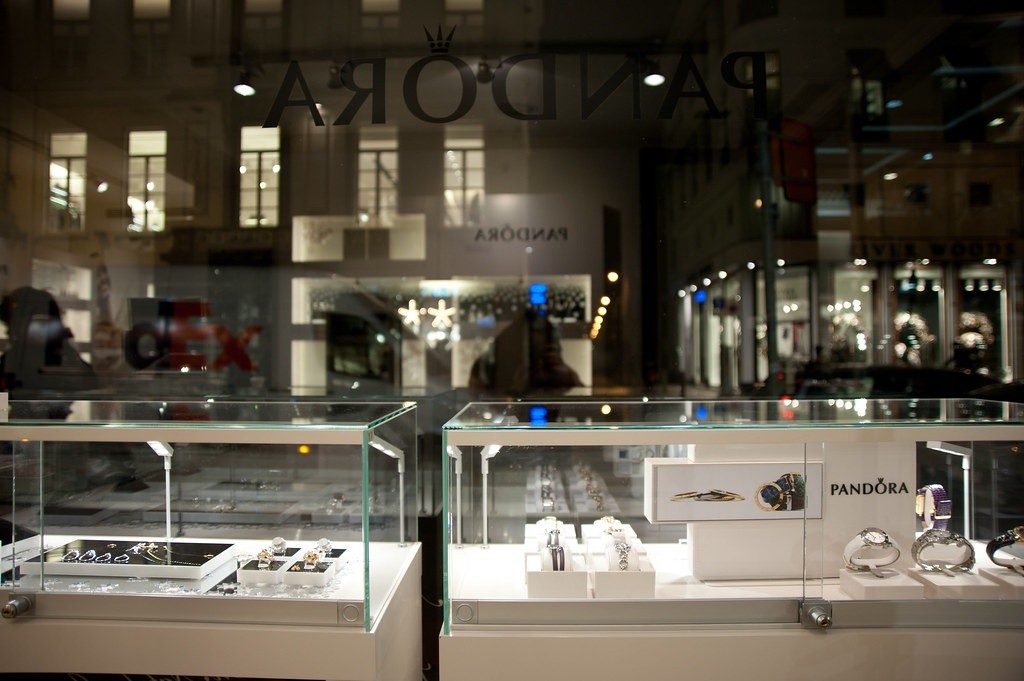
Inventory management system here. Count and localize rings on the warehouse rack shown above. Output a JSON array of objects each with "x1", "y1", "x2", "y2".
[{"x1": 62, "y1": 550, "x2": 129, "y2": 561}]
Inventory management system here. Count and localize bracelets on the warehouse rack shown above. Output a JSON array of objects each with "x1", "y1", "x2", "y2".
[
  {"x1": 579, "y1": 462, "x2": 604, "y2": 511},
  {"x1": 540, "y1": 464, "x2": 554, "y2": 511}
]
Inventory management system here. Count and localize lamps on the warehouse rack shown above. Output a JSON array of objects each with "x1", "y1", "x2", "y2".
[
  {"x1": 609, "y1": 41, "x2": 667, "y2": 102},
  {"x1": 224, "y1": 52, "x2": 274, "y2": 104}
]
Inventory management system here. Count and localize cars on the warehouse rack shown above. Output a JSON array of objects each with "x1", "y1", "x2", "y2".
[{"x1": 922, "y1": 380, "x2": 1024, "y2": 533}]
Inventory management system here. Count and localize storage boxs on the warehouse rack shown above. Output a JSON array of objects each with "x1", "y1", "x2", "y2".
[
  {"x1": 21, "y1": 540, "x2": 348, "y2": 589},
  {"x1": 523, "y1": 519, "x2": 657, "y2": 599}
]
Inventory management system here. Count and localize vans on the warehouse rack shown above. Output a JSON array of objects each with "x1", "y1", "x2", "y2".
[{"x1": 777, "y1": 364, "x2": 995, "y2": 431}]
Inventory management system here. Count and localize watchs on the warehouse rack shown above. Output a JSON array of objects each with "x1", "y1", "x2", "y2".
[
  {"x1": 916, "y1": 484, "x2": 952, "y2": 530},
  {"x1": 912, "y1": 529, "x2": 975, "y2": 573},
  {"x1": 545, "y1": 515, "x2": 565, "y2": 571},
  {"x1": 986, "y1": 527, "x2": 1024, "y2": 568},
  {"x1": 600, "y1": 515, "x2": 631, "y2": 570},
  {"x1": 844, "y1": 527, "x2": 900, "y2": 567}
]
